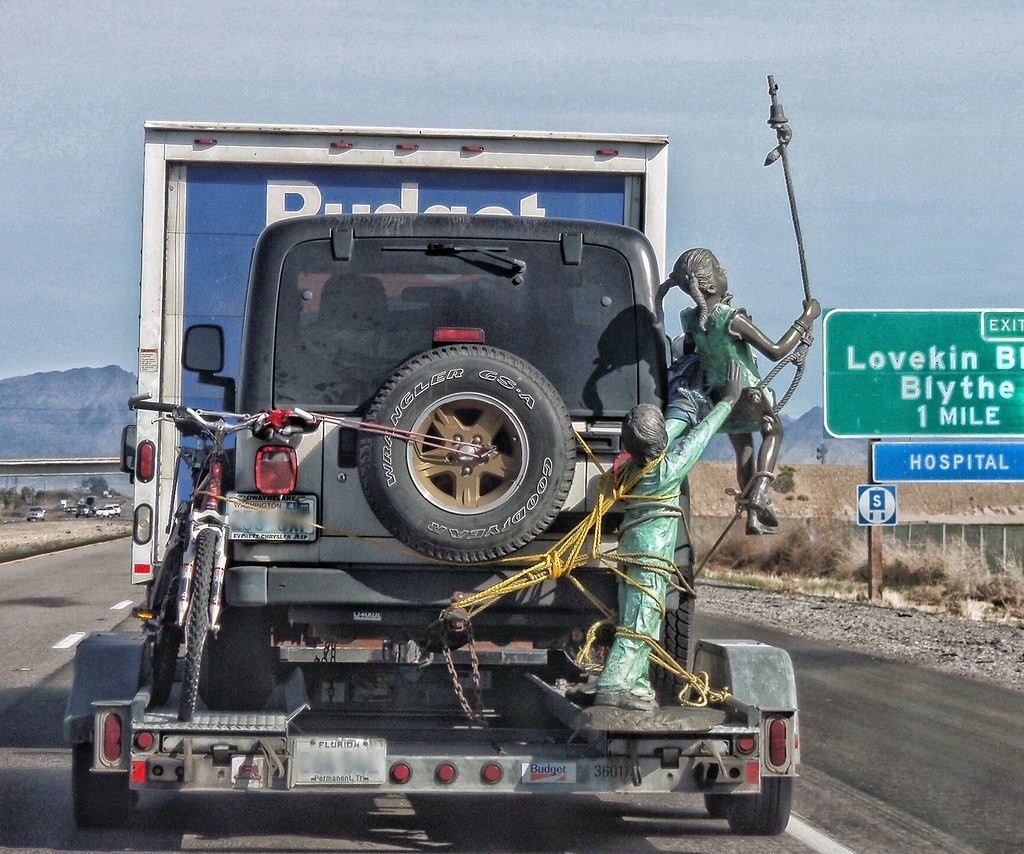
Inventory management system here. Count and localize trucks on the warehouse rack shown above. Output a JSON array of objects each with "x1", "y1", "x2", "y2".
[
  {"x1": 60, "y1": 500, "x2": 66, "y2": 510},
  {"x1": 85, "y1": 496, "x2": 97, "y2": 514}
]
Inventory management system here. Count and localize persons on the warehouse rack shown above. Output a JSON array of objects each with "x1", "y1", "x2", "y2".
[
  {"x1": 655, "y1": 248, "x2": 821, "y2": 535},
  {"x1": 592, "y1": 360, "x2": 743, "y2": 711}
]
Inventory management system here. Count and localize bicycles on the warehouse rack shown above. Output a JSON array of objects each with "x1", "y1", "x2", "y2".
[{"x1": 127, "y1": 394, "x2": 325, "y2": 723}]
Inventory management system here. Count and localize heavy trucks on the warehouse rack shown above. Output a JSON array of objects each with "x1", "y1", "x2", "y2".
[{"x1": 68, "y1": 121, "x2": 801, "y2": 836}]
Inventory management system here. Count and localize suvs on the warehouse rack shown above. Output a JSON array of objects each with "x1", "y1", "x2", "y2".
[{"x1": 223, "y1": 213, "x2": 696, "y2": 712}]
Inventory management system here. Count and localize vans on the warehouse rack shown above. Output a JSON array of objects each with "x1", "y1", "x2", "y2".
[{"x1": 75, "y1": 504, "x2": 90, "y2": 518}]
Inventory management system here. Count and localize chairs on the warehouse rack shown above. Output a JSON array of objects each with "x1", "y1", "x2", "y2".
[{"x1": 316, "y1": 273, "x2": 389, "y2": 329}]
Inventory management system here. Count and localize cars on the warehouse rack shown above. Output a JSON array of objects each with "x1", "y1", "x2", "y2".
[
  {"x1": 26, "y1": 507, "x2": 46, "y2": 521},
  {"x1": 96, "y1": 507, "x2": 111, "y2": 518},
  {"x1": 111, "y1": 504, "x2": 122, "y2": 517},
  {"x1": 64, "y1": 506, "x2": 76, "y2": 512},
  {"x1": 104, "y1": 505, "x2": 115, "y2": 517}
]
